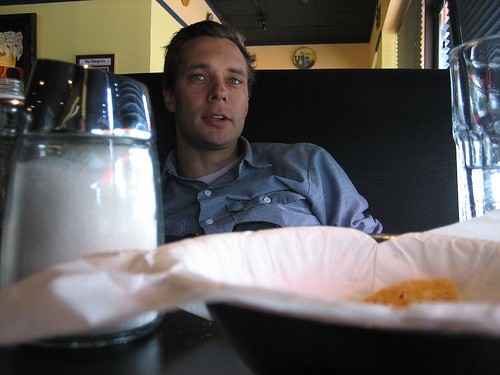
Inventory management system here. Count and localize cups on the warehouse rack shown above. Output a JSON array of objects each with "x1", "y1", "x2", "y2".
[{"x1": 446, "y1": 30, "x2": 499, "y2": 232}]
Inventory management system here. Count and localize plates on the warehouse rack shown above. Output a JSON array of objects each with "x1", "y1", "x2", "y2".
[{"x1": 201, "y1": 295, "x2": 500, "y2": 375}]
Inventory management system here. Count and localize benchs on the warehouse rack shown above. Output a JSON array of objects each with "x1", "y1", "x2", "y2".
[{"x1": 113, "y1": 67, "x2": 463, "y2": 234}]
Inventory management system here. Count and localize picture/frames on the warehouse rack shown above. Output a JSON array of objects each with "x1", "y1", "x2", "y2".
[
  {"x1": 0, "y1": 13, "x2": 38, "y2": 84},
  {"x1": 75, "y1": 54, "x2": 115, "y2": 74},
  {"x1": 291, "y1": 47, "x2": 316, "y2": 69}
]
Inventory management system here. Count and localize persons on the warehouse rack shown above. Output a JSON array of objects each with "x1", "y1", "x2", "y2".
[{"x1": 155, "y1": 17, "x2": 386, "y2": 242}]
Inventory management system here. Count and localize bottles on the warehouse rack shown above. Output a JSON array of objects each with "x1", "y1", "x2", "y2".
[{"x1": 0, "y1": 57, "x2": 172, "y2": 350}]
